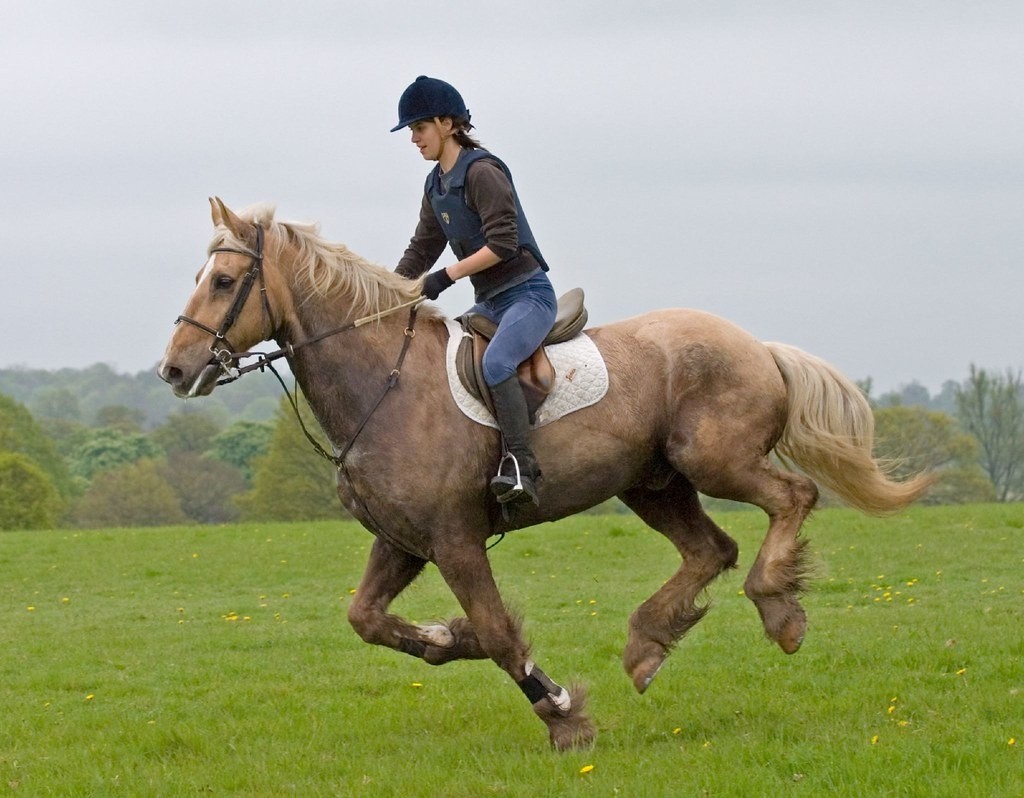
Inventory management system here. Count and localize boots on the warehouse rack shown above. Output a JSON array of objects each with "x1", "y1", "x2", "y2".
[{"x1": 490, "y1": 374, "x2": 540, "y2": 512}]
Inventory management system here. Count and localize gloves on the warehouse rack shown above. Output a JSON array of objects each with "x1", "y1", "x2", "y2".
[{"x1": 421, "y1": 268, "x2": 455, "y2": 300}]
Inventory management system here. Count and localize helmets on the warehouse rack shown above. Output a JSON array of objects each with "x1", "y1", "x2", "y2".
[{"x1": 390, "y1": 76, "x2": 472, "y2": 132}]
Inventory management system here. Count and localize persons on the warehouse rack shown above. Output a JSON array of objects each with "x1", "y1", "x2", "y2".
[{"x1": 392, "y1": 73, "x2": 558, "y2": 517}]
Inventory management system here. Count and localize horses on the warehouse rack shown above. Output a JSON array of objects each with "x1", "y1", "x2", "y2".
[{"x1": 156, "y1": 194, "x2": 940, "y2": 753}]
